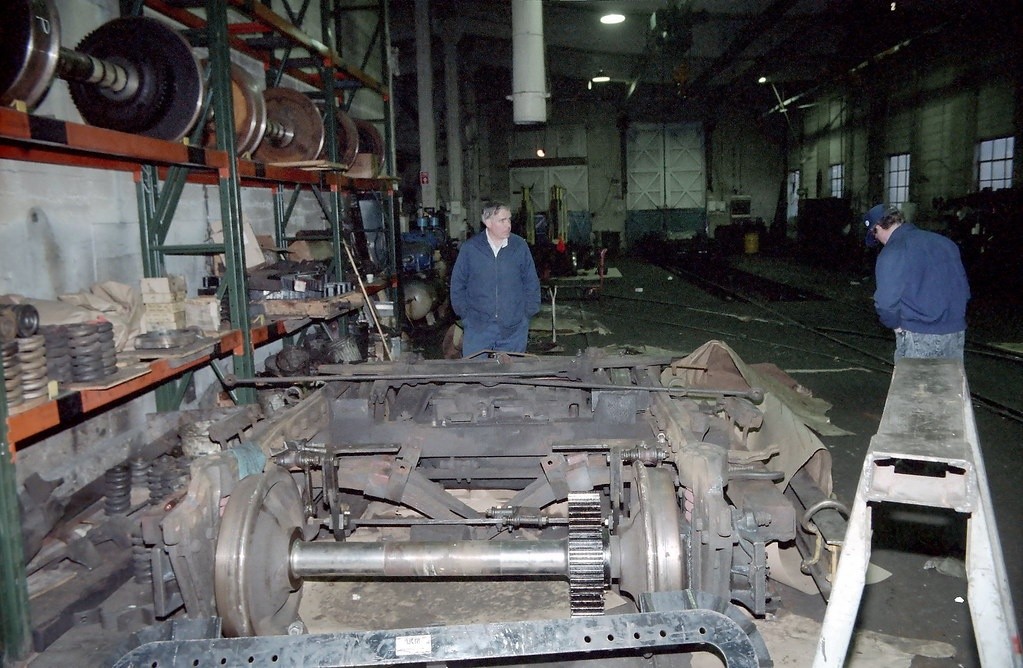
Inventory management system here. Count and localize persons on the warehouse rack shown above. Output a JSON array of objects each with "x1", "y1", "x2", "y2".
[
  {"x1": 864, "y1": 204, "x2": 971, "y2": 478},
  {"x1": 450, "y1": 201, "x2": 541, "y2": 358}
]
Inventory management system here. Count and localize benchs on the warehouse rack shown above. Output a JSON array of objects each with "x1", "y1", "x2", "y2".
[{"x1": 809, "y1": 354, "x2": 1023, "y2": 668}]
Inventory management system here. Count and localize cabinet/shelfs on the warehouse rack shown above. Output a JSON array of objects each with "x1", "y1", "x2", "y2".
[{"x1": 0, "y1": 0, "x2": 411, "y2": 668}]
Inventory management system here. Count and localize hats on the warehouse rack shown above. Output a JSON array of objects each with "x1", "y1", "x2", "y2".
[{"x1": 863, "y1": 202, "x2": 902, "y2": 246}]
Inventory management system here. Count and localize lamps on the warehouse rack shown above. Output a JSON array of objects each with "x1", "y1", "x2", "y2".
[
  {"x1": 599, "y1": 0, "x2": 627, "y2": 25},
  {"x1": 591, "y1": 66, "x2": 610, "y2": 83}
]
